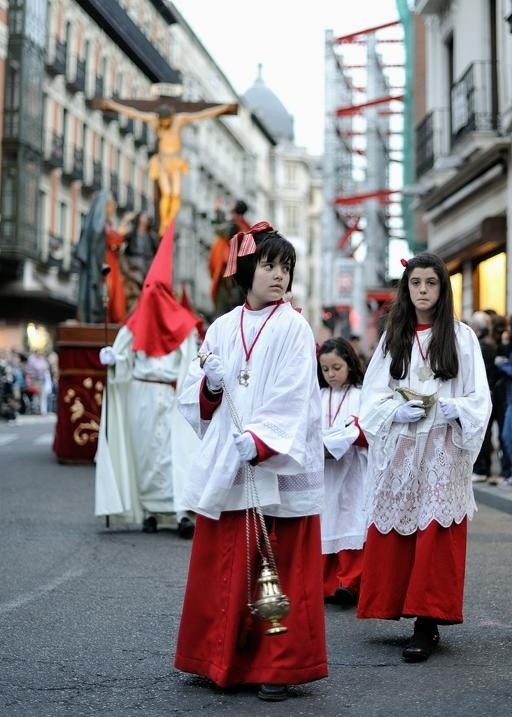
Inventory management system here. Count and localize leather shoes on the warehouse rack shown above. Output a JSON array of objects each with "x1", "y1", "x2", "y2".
[{"x1": 257, "y1": 683, "x2": 289, "y2": 702}]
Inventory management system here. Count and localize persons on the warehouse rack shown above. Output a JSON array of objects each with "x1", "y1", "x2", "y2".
[
  {"x1": 317, "y1": 336, "x2": 373, "y2": 597},
  {"x1": 174, "y1": 222, "x2": 329, "y2": 697},
  {"x1": 358, "y1": 253, "x2": 493, "y2": 663},
  {"x1": 100, "y1": 100, "x2": 231, "y2": 237},
  {"x1": 466, "y1": 309, "x2": 512, "y2": 482},
  {"x1": 96, "y1": 273, "x2": 207, "y2": 538},
  {"x1": 0, "y1": 343, "x2": 60, "y2": 422}
]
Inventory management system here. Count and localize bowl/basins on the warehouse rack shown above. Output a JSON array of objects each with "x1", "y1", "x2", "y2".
[{"x1": 391, "y1": 384, "x2": 441, "y2": 409}]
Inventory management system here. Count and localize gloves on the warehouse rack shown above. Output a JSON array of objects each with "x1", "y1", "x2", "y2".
[
  {"x1": 202, "y1": 354, "x2": 225, "y2": 392},
  {"x1": 439, "y1": 397, "x2": 459, "y2": 420},
  {"x1": 232, "y1": 430, "x2": 258, "y2": 463},
  {"x1": 393, "y1": 399, "x2": 426, "y2": 424},
  {"x1": 99, "y1": 347, "x2": 116, "y2": 367}
]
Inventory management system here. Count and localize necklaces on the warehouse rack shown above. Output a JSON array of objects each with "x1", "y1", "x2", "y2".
[
  {"x1": 415, "y1": 332, "x2": 432, "y2": 383},
  {"x1": 238, "y1": 297, "x2": 282, "y2": 386}
]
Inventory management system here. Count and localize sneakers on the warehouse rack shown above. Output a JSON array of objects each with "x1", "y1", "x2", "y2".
[
  {"x1": 142, "y1": 516, "x2": 156, "y2": 532},
  {"x1": 472, "y1": 474, "x2": 511, "y2": 490},
  {"x1": 402, "y1": 620, "x2": 439, "y2": 663},
  {"x1": 179, "y1": 516, "x2": 194, "y2": 539},
  {"x1": 335, "y1": 588, "x2": 355, "y2": 610}
]
